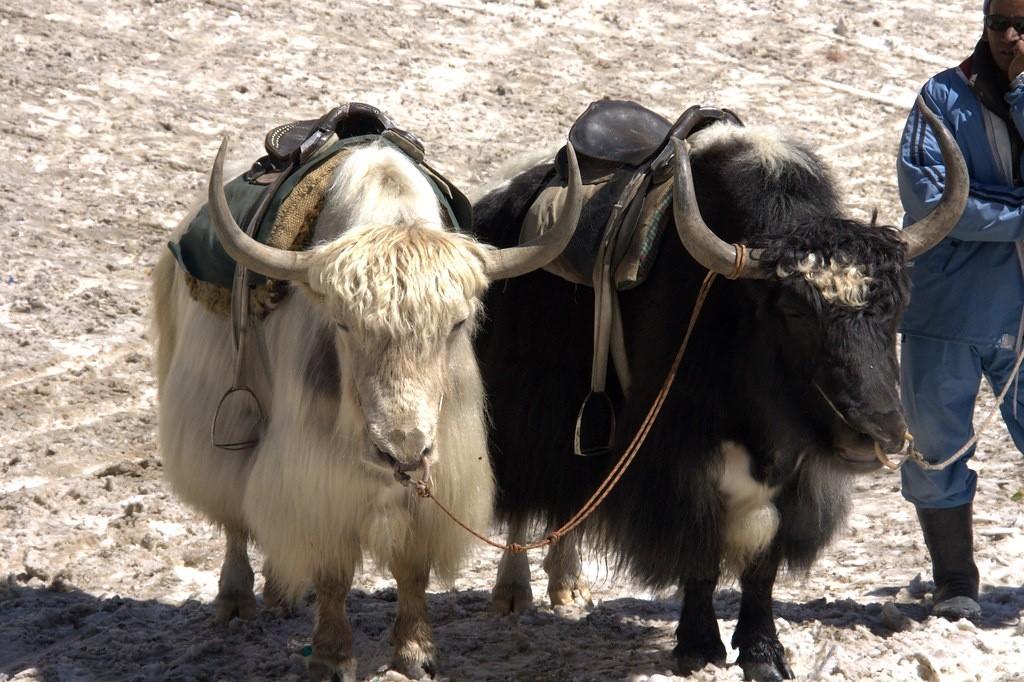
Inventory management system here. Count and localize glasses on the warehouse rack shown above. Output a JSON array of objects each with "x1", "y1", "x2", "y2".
[{"x1": 984, "y1": 15, "x2": 1024, "y2": 34}]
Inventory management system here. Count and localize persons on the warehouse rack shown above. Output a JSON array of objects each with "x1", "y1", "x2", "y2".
[{"x1": 898, "y1": 1, "x2": 1024, "y2": 621}]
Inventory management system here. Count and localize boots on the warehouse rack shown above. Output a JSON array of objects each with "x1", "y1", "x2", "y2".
[{"x1": 917, "y1": 503, "x2": 982, "y2": 620}]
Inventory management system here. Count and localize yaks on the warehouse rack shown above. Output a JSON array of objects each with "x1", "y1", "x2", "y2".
[{"x1": 154, "y1": 93, "x2": 971, "y2": 682}]
